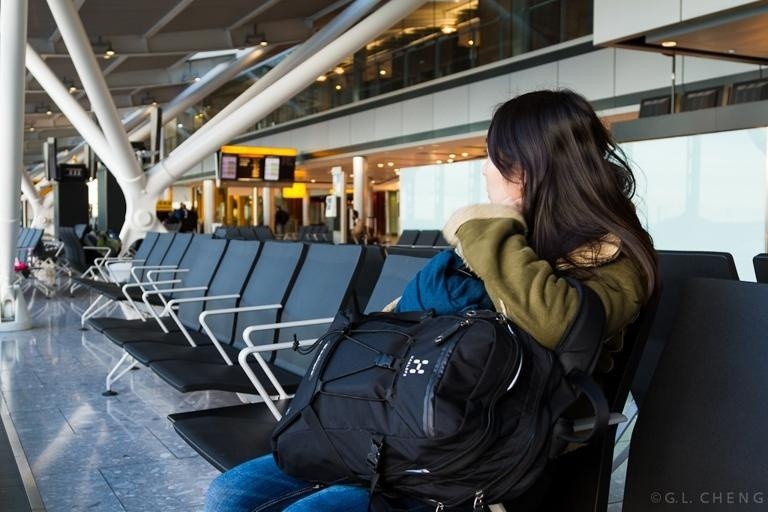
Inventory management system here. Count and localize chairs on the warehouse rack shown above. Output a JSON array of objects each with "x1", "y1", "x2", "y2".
[{"x1": 15, "y1": 219, "x2": 767, "y2": 511}]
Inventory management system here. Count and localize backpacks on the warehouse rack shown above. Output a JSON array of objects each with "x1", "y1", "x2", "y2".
[{"x1": 270, "y1": 310, "x2": 610, "y2": 511}]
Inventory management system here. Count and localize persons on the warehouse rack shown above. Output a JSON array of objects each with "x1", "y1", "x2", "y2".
[
  {"x1": 275, "y1": 204, "x2": 289, "y2": 239},
  {"x1": 201, "y1": 89, "x2": 660, "y2": 510},
  {"x1": 164, "y1": 203, "x2": 190, "y2": 226},
  {"x1": 352, "y1": 208, "x2": 363, "y2": 240}
]
